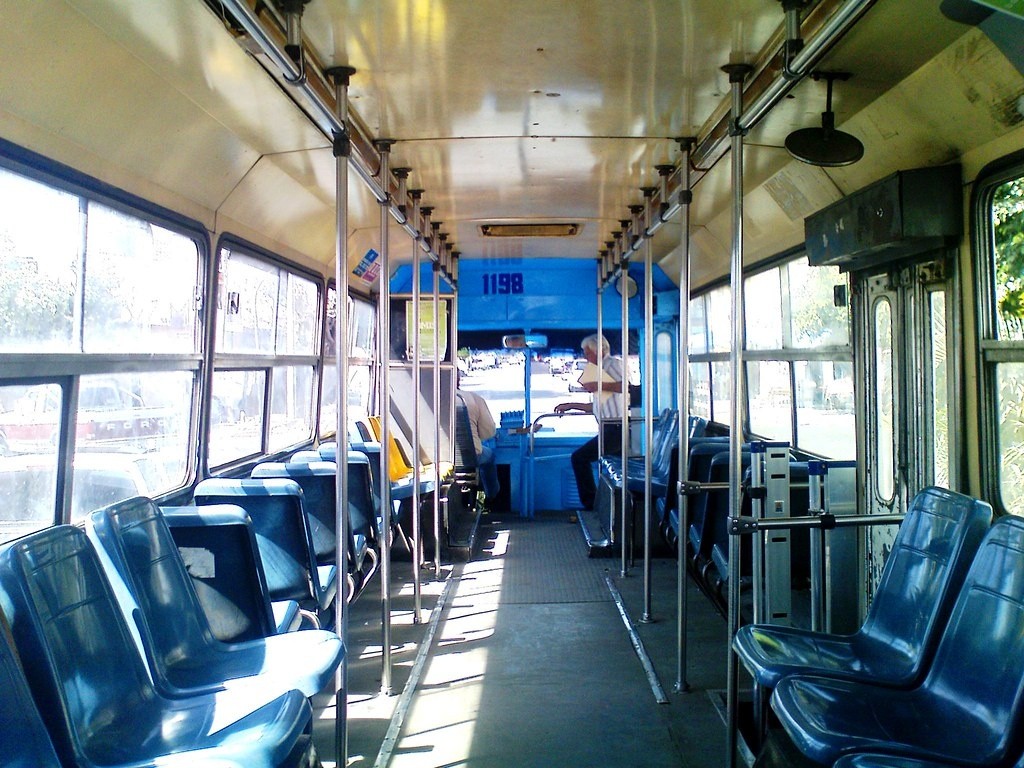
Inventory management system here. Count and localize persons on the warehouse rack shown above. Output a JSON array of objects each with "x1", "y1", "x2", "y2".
[
  {"x1": 457, "y1": 366, "x2": 500, "y2": 499},
  {"x1": 554, "y1": 335, "x2": 631, "y2": 522},
  {"x1": 506, "y1": 337, "x2": 528, "y2": 347}
]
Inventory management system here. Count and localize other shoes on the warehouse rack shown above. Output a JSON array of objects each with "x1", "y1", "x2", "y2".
[{"x1": 570, "y1": 515, "x2": 578, "y2": 523}]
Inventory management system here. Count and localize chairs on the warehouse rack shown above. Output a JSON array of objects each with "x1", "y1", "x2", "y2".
[
  {"x1": 454, "y1": 394, "x2": 492, "y2": 515},
  {"x1": 655, "y1": 437, "x2": 819, "y2": 638},
  {"x1": 158, "y1": 438, "x2": 398, "y2": 737}
]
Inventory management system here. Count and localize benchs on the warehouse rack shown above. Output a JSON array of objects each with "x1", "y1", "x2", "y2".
[
  {"x1": 347, "y1": 414, "x2": 454, "y2": 569},
  {"x1": 733, "y1": 487, "x2": 1024, "y2": 768},
  {"x1": 0, "y1": 497, "x2": 345, "y2": 768},
  {"x1": 597, "y1": 408, "x2": 705, "y2": 566}
]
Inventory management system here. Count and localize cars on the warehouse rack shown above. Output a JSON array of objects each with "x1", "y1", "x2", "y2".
[
  {"x1": 828, "y1": 376, "x2": 856, "y2": 410},
  {"x1": 561, "y1": 361, "x2": 574, "y2": 380},
  {"x1": 531, "y1": 351, "x2": 551, "y2": 364},
  {"x1": 0, "y1": 373, "x2": 188, "y2": 542},
  {"x1": 457, "y1": 352, "x2": 525, "y2": 377},
  {"x1": 567, "y1": 359, "x2": 592, "y2": 393},
  {"x1": 141, "y1": 372, "x2": 242, "y2": 420}
]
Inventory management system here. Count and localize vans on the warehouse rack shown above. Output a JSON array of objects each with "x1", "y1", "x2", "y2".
[{"x1": 550, "y1": 348, "x2": 575, "y2": 377}]
